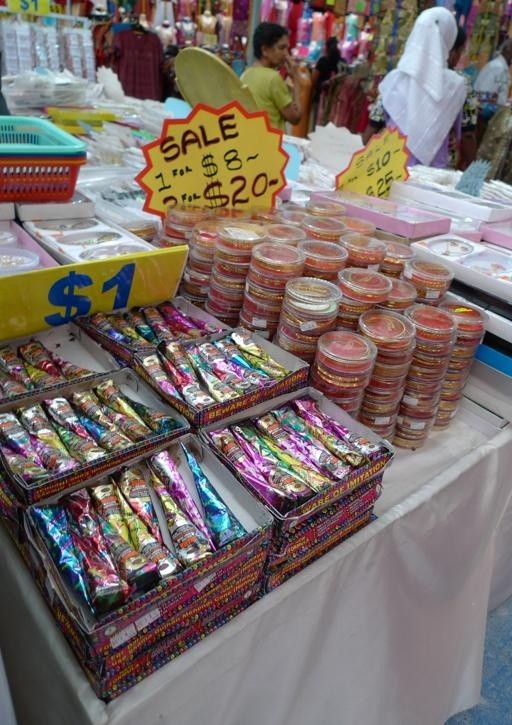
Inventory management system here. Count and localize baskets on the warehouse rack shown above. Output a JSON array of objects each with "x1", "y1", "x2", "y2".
[{"x1": 0, "y1": 113, "x2": 89, "y2": 203}]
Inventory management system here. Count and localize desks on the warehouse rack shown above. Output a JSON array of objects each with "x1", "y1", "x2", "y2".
[{"x1": 0, "y1": 88, "x2": 511, "y2": 720}]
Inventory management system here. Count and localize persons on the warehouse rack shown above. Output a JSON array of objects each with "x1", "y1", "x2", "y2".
[
  {"x1": 311, "y1": 34, "x2": 349, "y2": 98},
  {"x1": 375, "y1": 6, "x2": 474, "y2": 171},
  {"x1": 237, "y1": 21, "x2": 303, "y2": 134},
  {"x1": 475, "y1": 39, "x2": 512, "y2": 127}
]
